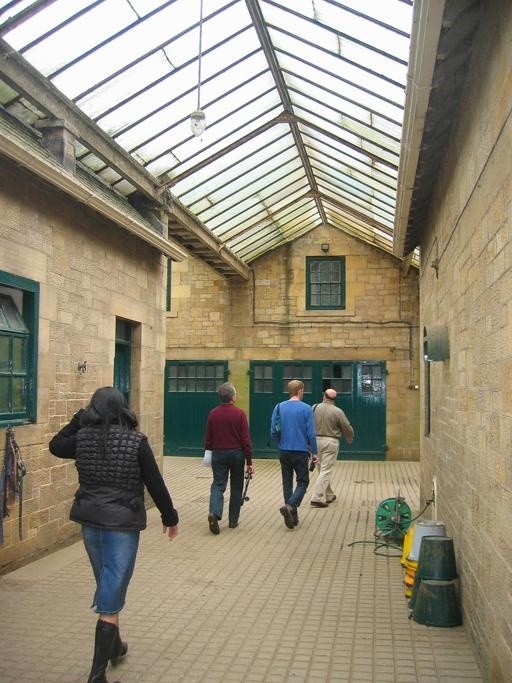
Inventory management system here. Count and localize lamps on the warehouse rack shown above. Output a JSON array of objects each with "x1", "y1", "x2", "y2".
[
  {"x1": 187, "y1": 0, "x2": 212, "y2": 138},
  {"x1": 320, "y1": 242, "x2": 330, "y2": 252}
]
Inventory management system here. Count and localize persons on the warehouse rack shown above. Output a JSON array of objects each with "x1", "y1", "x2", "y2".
[
  {"x1": 310, "y1": 388, "x2": 354, "y2": 508},
  {"x1": 205, "y1": 382, "x2": 253, "y2": 535},
  {"x1": 269, "y1": 381, "x2": 316, "y2": 528},
  {"x1": 47, "y1": 387, "x2": 179, "y2": 682}
]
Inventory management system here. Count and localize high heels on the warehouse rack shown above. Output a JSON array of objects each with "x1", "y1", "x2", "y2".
[{"x1": 110, "y1": 636, "x2": 128, "y2": 668}]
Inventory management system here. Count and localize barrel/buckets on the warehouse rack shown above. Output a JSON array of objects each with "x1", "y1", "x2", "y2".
[{"x1": 400, "y1": 520, "x2": 460, "y2": 627}]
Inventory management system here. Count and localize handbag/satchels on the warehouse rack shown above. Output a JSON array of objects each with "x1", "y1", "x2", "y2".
[{"x1": 274, "y1": 404, "x2": 281, "y2": 441}]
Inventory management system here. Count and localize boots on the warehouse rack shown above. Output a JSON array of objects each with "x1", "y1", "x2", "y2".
[{"x1": 86, "y1": 619, "x2": 119, "y2": 682}]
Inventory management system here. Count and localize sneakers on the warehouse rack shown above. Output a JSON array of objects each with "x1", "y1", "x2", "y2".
[
  {"x1": 280, "y1": 506, "x2": 295, "y2": 529},
  {"x1": 229, "y1": 521, "x2": 240, "y2": 528},
  {"x1": 291, "y1": 506, "x2": 297, "y2": 526},
  {"x1": 310, "y1": 500, "x2": 329, "y2": 508},
  {"x1": 327, "y1": 494, "x2": 337, "y2": 503},
  {"x1": 207, "y1": 512, "x2": 220, "y2": 534}
]
